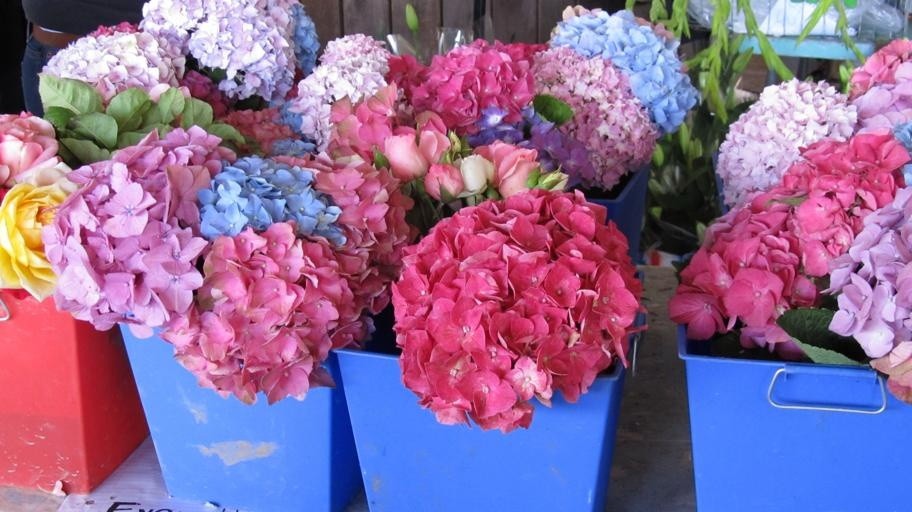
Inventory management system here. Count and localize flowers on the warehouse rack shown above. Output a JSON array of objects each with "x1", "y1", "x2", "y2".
[
  {"x1": 38, "y1": 22, "x2": 246, "y2": 168},
  {"x1": 846, "y1": 38, "x2": 912, "y2": 117},
  {"x1": 47, "y1": 127, "x2": 420, "y2": 407},
  {"x1": 672, "y1": 119, "x2": 912, "y2": 406},
  {"x1": 423, "y1": 42, "x2": 531, "y2": 148},
  {"x1": 715, "y1": 76, "x2": 857, "y2": 207},
  {"x1": 0, "y1": 110, "x2": 82, "y2": 303},
  {"x1": 392, "y1": 188, "x2": 650, "y2": 433},
  {"x1": 299, "y1": 30, "x2": 423, "y2": 148},
  {"x1": 141, "y1": 0, "x2": 320, "y2": 112},
  {"x1": 535, "y1": 0, "x2": 698, "y2": 192}
]
